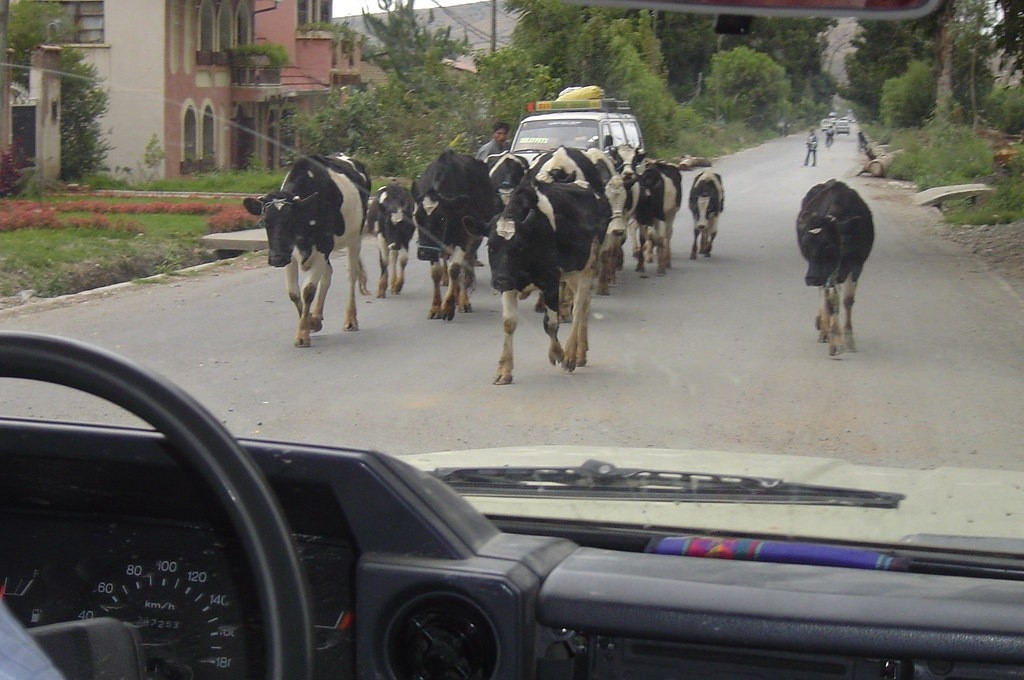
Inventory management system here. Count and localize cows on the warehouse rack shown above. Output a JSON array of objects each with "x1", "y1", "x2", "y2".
[
  {"x1": 241, "y1": 143, "x2": 726, "y2": 386},
  {"x1": 795, "y1": 178, "x2": 875, "y2": 356}
]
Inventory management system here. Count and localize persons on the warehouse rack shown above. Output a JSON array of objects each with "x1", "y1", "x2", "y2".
[
  {"x1": 804, "y1": 127, "x2": 817, "y2": 166},
  {"x1": 826, "y1": 125, "x2": 834, "y2": 148},
  {"x1": 476, "y1": 123, "x2": 511, "y2": 172},
  {"x1": 858, "y1": 131, "x2": 867, "y2": 150}
]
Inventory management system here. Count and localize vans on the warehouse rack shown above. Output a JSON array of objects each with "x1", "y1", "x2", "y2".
[
  {"x1": 509, "y1": 112, "x2": 647, "y2": 169},
  {"x1": 836, "y1": 121, "x2": 849, "y2": 135}
]
